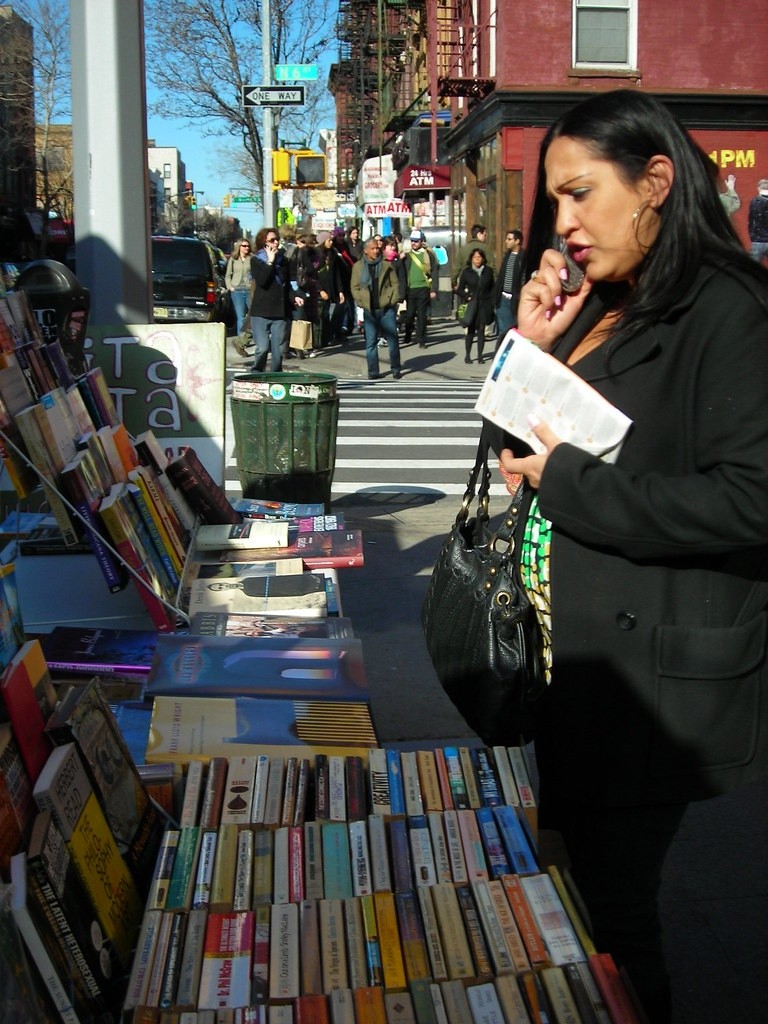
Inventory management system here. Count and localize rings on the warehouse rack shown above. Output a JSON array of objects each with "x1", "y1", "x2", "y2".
[{"x1": 531, "y1": 271, "x2": 543, "y2": 285}]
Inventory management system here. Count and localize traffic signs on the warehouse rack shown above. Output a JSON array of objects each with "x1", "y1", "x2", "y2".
[{"x1": 242, "y1": 84, "x2": 306, "y2": 108}]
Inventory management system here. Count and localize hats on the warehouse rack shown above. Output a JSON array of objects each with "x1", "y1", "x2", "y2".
[
  {"x1": 316, "y1": 232, "x2": 331, "y2": 245},
  {"x1": 333, "y1": 227, "x2": 345, "y2": 237},
  {"x1": 410, "y1": 230, "x2": 425, "y2": 241}
]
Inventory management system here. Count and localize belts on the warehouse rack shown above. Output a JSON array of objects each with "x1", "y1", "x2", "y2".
[{"x1": 501, "y1": 292, "x2": 512, "y2": 301}]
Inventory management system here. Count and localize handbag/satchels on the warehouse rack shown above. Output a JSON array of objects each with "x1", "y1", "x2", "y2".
[
  {"x1": 461, "y1": 301, "x2": 477, "y2": 328},
  {"x1": 424, "y1": 272, "x2": 432, "y2": 287},
  {"x1": 296, "y1": 266, "x2": 309, "y2": 287},
  {"x1": 419, "y1": 432, "x2": 549, "y2": 747},
  {"x1": 289, "y1": 304, "x2": 313, "y2": 350}
]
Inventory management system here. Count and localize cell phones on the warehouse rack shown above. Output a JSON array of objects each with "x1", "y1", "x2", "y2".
[{"x1": 560, "y1": 244, "x2": 586, "y2": 292}]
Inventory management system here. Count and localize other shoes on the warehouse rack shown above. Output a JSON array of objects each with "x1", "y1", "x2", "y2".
[
  {"x1": 420, "y1": 344, "x2": 427, "y2": 349},
  {"x1": 478, "y1": 358, "x2": 485, "y2": 364},
  {"x1": 465, "y1": 358, "x2": 473, "y2": 364},
  {"x1": 304, "y1": 349, "x2": 326, "y2": 358},
  {"x1": 404, "y1": 333, "x2": 411, "y2": 343},
  {"x1": 294, "y1": 350, "x2": 306, "y2": 359},
  {"x1": 283, "y1": 352, "x2": 295, "y2": 360},
  {"x1": 231, "y1": 339, "x2": 248, "y2": 356}
]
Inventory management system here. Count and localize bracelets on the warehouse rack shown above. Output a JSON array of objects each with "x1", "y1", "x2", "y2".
[{"x1": 267, "y1": 260, "x2": 272, "y2": 264}]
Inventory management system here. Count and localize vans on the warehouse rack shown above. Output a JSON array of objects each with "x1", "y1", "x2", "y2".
[{"x1": 152, "y1": 233, "x2": 230, "y2": 323}]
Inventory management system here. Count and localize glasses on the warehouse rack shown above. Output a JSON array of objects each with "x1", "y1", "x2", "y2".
[
  {"x1": 411, "y1": 240, "x2": 418, "y2": 242},
  {"x1": 241, "y1": 245, "x2": 249, "y2": 247},
  {"x1": 266, "y1": 238, "x2": 279, "y2": 243}
]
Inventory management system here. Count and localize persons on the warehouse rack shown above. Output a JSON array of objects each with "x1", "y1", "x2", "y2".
[
  {"x1": 189, "y1": 222, "x2": 529, "y2": 380},
  {"x1": 486, "y1": 88, "x2": 768, "y2": 1024},
  {"x1": 717, "y1": 172, "x2": 768, "y2": 266},
  {"x1": 205, "y1": 573, "x2": 325, "y2": 598}
]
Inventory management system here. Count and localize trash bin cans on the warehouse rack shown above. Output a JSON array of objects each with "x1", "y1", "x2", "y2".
[{"x1": 231, "y1": 368, "x2": 343, "y2": 516}]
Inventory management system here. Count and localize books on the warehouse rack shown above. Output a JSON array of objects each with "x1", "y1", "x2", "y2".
[{"x1": 0, "y1": 289, "x2": 643, "y2": 1024}]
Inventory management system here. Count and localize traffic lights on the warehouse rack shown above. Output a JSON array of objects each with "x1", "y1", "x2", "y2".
[
  {"x1": 191, "y1": 197, "x2": 196, "y2": 211},
  {"x1": 294, "y1": 155, "x2": 327, "y2": 187}
]
